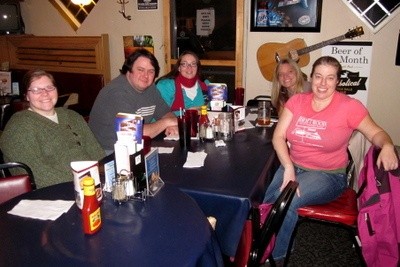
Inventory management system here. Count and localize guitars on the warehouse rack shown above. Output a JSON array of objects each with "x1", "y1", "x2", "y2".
[{"x1": 256, "y1": 25, "x2": 365, "y2": 82}]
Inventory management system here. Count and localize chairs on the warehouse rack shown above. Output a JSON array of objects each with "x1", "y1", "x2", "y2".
[
  {"x1": 233, "y1": 180, "x2": 300, "y2": 267},
  {"x1": 283, "y1": 131, "x2": 366, "y2": 267},
  {"x1": 0, "y1": 163, "x2": 37, "y2": 205}
]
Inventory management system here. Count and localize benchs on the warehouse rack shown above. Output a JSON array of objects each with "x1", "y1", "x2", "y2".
[{"x1": 9, "y1": 69, "x2": 107, "y2": 127}]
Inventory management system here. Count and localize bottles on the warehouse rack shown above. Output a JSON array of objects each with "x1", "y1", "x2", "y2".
[
  {"x1": 198, "y1": 106, "x2": 209, "y2": 143},
  {"x1": 111, "y1": 173, "x2": 136, "y2": 205},
  {"x1": 81, "y1": 177, "x2": 102, "y2": 234}
]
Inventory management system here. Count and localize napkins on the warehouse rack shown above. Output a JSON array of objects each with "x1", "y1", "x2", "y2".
[
  {"x1": 234, "y1": 113, "x2": 277, "y2": 131},
  {"x1": 151, "y1": 147, "x2": 175, "y2": 154},
  {"x1": 215, "y1": 139, "x2": 227, "y2": 147},
  {"x1": 7, "y1": 199, "x2": 75, "y2": 220},
  {"x1": 164, "y1": 134, "x2": 178, "y2": 141},
  {"x1": 184, "y1": 151, "x2": 207, "y2": 168}
]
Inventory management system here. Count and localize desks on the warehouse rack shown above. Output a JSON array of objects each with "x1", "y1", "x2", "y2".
[
  {"x1": 99, "y1": 118, "x2": 277, "y2": 267},
  {"x1": 0, "y1": 181, "x2": 227, "y2": 267}
]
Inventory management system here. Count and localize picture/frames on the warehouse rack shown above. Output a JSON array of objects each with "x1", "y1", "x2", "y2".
[{"x1": 250, "y1": 0, "x2": 324, "y2": 32}]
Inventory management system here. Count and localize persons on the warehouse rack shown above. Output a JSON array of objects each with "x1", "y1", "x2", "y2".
[
  {"x1": 271, "y1": 59, "x2": 312, "y2": 120},
  {"x1": 0, "y1": 67, "x2": 106, "y2": 190},
  {"x1": 150, "y1": 50, "x2": 211, "y2": 124},
  {"x1": 88, "y1": 48, "x2": 179, "y2": 150},
  {"x1": 264, "y1": 56, "x2": 399, "y2": 262}
]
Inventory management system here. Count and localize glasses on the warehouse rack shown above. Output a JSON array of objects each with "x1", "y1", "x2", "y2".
[
  {"x1": 180, "y1": 62, "x2": 198, "y2": 67},
  {"x1": 26, "y1": 86, "x2": 56, "y2": 95}
]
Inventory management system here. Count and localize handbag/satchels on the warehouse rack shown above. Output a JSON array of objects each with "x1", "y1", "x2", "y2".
[{"x1": 259, "y1": 202, "x2": 275, "y2": 263}]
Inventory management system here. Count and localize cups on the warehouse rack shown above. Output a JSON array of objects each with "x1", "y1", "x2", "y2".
[
  {"x1": 184, "y1": 108, "x2": 198, "y2": 137},
  {"x1": 258, "y1": 102, "x2": 271, "y2": 125},
  {"x1": 178, "y1": 114, "x2": 191, "y2": 153},
  {"x1": 217, "y1": 112, "x2": 233, "y2": 141}
]
